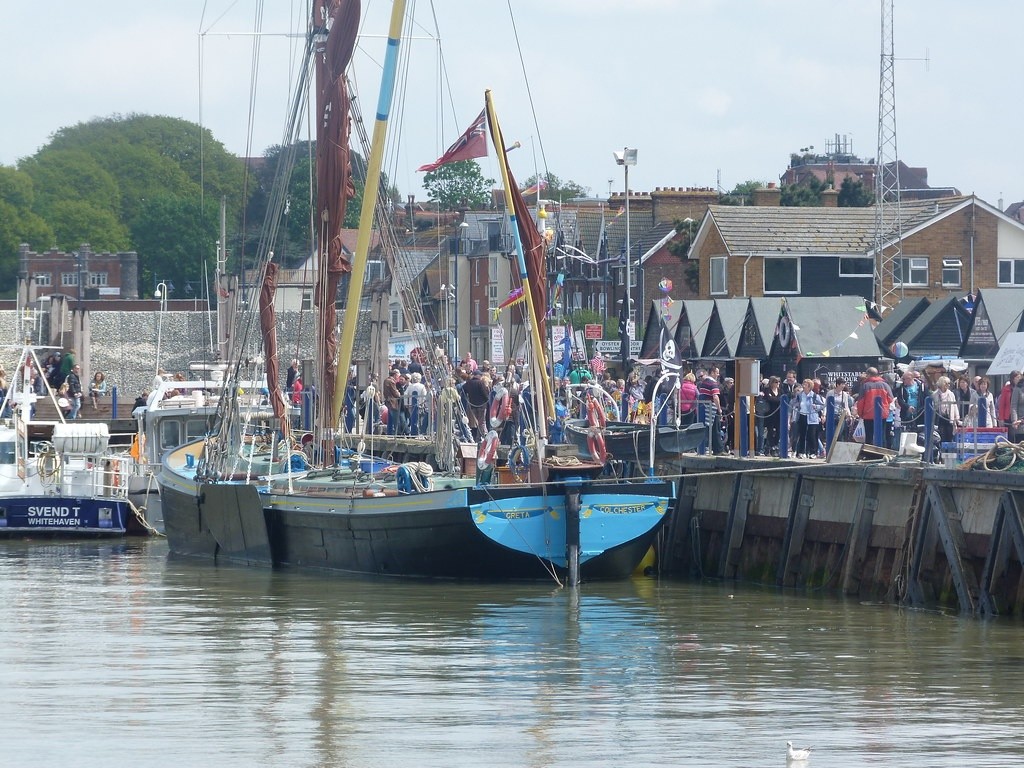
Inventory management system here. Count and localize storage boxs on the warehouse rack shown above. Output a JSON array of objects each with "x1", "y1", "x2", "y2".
[
  {"x1": 957, "y1": 443, "x2": 997, "y2": 460},
  {"x1": 350, "y1": 460, "x2": 390, "y2": 473},
  {"x1": 956, "y1": 428, "x2": 1008, "y2": 443}
]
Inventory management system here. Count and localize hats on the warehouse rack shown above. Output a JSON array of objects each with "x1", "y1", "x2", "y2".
[
  {"x1": 474, "y1": 370, "x2": 483, "y2": 376},
  {"x1": 55, "y1": 352, "x2": 60, "y2": 356}
]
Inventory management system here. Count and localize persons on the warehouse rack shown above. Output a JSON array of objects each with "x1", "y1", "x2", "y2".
[
  {"x1": 131, "y1": 369, "x2": 187, "y2": 432},
  {"x1": 523, "y1": 352, "x2": 664, "y2": 445},
  {"x1": 343, "y1": 357, "x2": 441, "y2": 435},
  {"x1": 435, "y1": 352, "x2": 524, "y2": 442},
  {"x1": 0, "y1": 349, "x2": 107, "y2": 420},
  {"x1": 923, "y1": 361, "x2": 1024, "y2": 452},
  {"x1": 681, "y1": 365, "x2": 928, "y2": 458},
  {"x1": 287, "y1": 359, "x2": 317, "y2": 407}
]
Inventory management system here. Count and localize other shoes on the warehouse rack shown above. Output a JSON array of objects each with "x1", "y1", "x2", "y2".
[
  {"x1": 809, "y1": 454, "x2": 816, "y2": 459},
  {"x1": 713, "y1": 445, "x2": 797, "y2": 458},
  {"x1": 799, "y1": 453, "x2": 806, "y2": 459},
  {"x1": 817, "y1": 450, "x2": 826, "y2": 458}
]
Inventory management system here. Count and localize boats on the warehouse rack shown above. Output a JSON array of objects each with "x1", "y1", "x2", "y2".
[
  {"x1": 114, "y1": 361, "x2": 300, "y2": 535},
  {"x1": 0, "y1": 339, "x2": 136, "y2": 534}
]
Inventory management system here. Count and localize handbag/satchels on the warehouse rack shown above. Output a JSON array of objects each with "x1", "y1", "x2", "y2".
[
  {"x1": 836, "y1": 401, "x2": 846, "y2": 422},
  {"x1": 853, "y1": 417, "x2": 866, "y2": 442}
]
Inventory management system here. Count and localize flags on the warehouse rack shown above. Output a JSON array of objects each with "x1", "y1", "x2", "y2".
[{"x1": 416, "y1": 107, "x2": 487, "y2": 172}]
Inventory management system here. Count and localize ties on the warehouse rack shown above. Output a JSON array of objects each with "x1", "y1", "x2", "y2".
[{"x1": 789, "y1": 384, "x2": 793, "y2": 392}]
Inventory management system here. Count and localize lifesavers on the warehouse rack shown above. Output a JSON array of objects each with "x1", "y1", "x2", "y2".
[
  {"x1": 489, "y1": 387, "x2": 509, "y2": 428},
  {"x1": 477, "y1": 429, "x2": 498, "y2": 469},
  {"x1": 113, "y1": 460, "x2": 119, "y2": 486},
  {"x1": 585, "y1": 395, "x2": 606, "y2": 430},
  {"x1": 587, "y1": 426, "x2": 606, "y2": 465},
  {"x1": 404, "y1": 382, "x2": 427, "y2": 408}
]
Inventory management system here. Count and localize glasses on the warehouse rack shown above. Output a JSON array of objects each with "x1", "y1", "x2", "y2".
[
  {"x1": 480, "y1": 374, "x2": 482, "y2": 376},
  {"x1": 697, "y1": 374, "x2": 705, "y2": 379},
  {"x1": 729, "y1": 382, "x2": 734, "y2": 384},
  {"x1": 902, "y1": 378, "x2": 910, "y2": 380},
  {"x1": 773, "y1": 381, "x2": 780, "y2": 383},
  {"x1": 914, "y1": 376, "x2": 920, "y2": 378}
]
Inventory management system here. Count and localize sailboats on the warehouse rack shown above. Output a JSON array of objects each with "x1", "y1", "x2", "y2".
[{"x1": 156, "y1": 0, "x2": 679, "y2": 589}]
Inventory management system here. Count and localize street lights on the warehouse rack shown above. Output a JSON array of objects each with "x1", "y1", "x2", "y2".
[
  {"x1": 613, "y1": 146, "x2": 639, "y2": 389},
  {"x1": 453, "y1": 217, "x2": 471, "y2": 372},
  {"x1": 601, "y1": 230, "x2": 608, "y2": 343}
]
